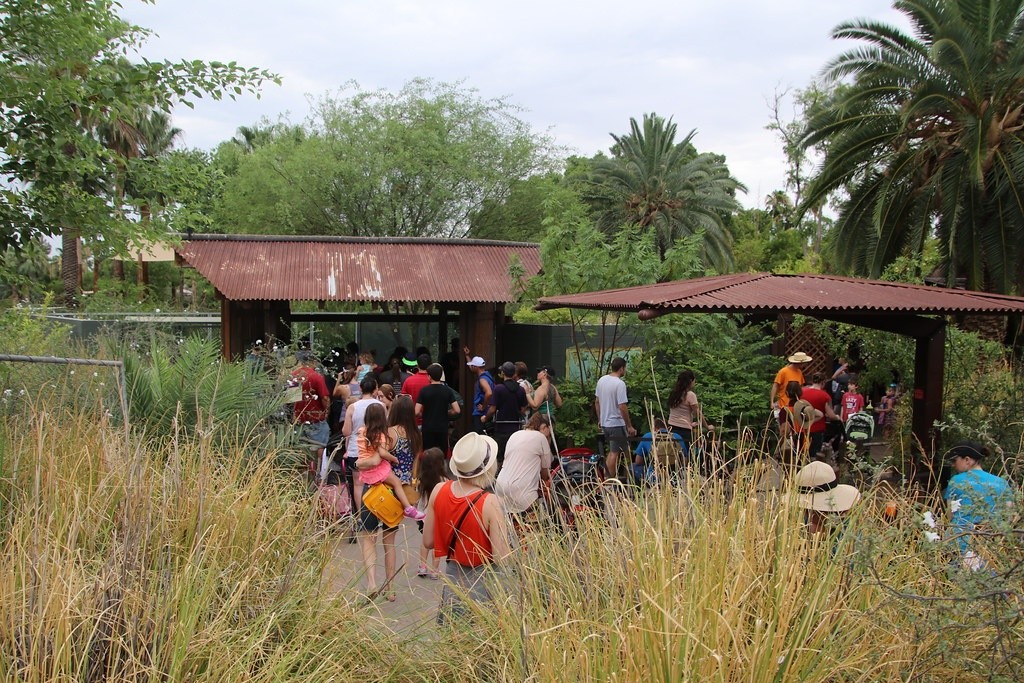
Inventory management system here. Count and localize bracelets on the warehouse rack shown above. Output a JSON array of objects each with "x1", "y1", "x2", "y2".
[
  {"x1": 355, "y1": 463, "x2": 356, "y2": 467},
  {"x1": 465, "y1": 354, "x2": 469, "y2": 356}
]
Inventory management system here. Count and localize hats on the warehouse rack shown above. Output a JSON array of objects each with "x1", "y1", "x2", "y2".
[
  {"x1": 788, "y1": 352, "x2": 812, "y2": 363},
  {"x1": 782, "y1": 461, "x2": 861, "y2": 512},
  {"x1": 466, "y1": 356, "x2": 486, "y2": 366},
  {"x1": 536, "y1": 365, "x2": 555, "y2": 377},
  {"x1": 295, "y1": 350, "x2": 321, "y2": 362},
  {"x1": 794, "y1": 399, "x2": 815, "y2": 429},
  {"x1": 402, "y1": 353, "x2": 418, "y2": 366},
  {"x1": 942, "y1": 441, "x2": 985, "y2": 460},
  {"x1": 449, "y1": 432, "x2": 499, "y2": 478},
  {"x1": 499, "y1": 361, "x2": 516, "y2": 375}
]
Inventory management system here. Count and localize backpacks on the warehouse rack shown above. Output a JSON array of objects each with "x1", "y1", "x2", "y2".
[{"x1": 651, "y1": 434, "x2": 682, "y2": 466}]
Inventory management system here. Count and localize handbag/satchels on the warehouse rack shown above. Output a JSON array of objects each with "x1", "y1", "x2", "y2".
[
  {"x1": 364, "y1": 484, "x2": 405, "y2": 529},
  {"x1": 320, "y1": 483, "x2": 352, "y2": 517}
]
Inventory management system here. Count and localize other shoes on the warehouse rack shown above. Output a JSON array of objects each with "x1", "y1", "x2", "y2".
[
  {"x1": 416, "y1": 565, "x2": 428, "y2": 576},
  {"x1": 382, "y1": 590, "x2": 398, "y2": 602},
  {"x1": 431, "y1": 568, "x2": 440, "y2": 580},
  {"x1": 348, "y1": 535, "x2": 357, "y2": 545},
  {"x1": 366, "y1": 590, "x2": 378, "y2": 604}
]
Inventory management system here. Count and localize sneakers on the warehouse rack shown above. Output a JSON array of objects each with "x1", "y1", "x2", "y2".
[{"x1": 403, "y1": 505, "x2": 426, "y2": 520}]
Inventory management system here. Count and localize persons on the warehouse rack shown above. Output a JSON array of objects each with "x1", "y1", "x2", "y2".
[
  {"x1": 877, "y1": 384, "x2": 898, "y2": 441},
  {"x1": 782, "y1": 460, "x2": 861, "y2": 542},
  {"x1": 771, "y1": 352, "x2": 813, "y2": 458},
  {"x1": 840, "y1": 376, "x2": 865, "y2": 424},
  {"x1": 595, "y1": 357, "x2": 637, "y2": 476},
  {"x1": 947, "y1": 442, "x2": 1015, "y2": 577},
  {"x1": 354, "y1": 394, "x2": 421, "y2": 602},
  {"x1": 800, "y1": 371, "x2": 836, "y2": 457},
  {"x1": 668, "y1": 370, "x2": 714, "y2": 451},
  {"x1": 248, "y1": 338, "x2": 562, "y2": 546},
  {"x1": 779, "y1": 381, "x2": 806, "y2": 466},
  {"x1": 416, "y1": 448, "x2": 451, "y2": 579},
  {"x1": 496, "y1": 412, "x2": 555, "y2": 518},
  {"x1": 630, "y1": 418, "x2": 688, "y2": 488},
  {"x1": 423, "y1": 431, "x2": 517, "y2": 633},
  {"x1": 831, "y1": 361, "x2": 859, "y2": 415},
  {"x1": 354, "y1": 403, "x2": 426, "y2": 525}
]
